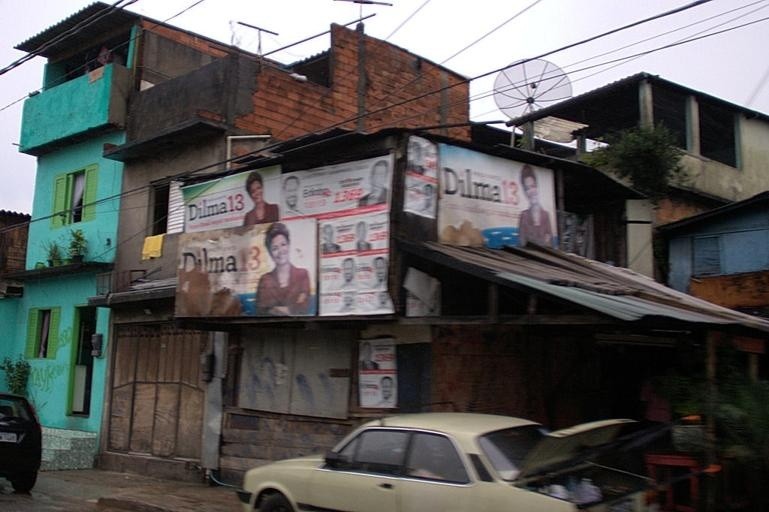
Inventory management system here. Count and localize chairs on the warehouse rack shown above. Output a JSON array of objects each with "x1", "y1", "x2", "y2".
[
  {"x1": 0, "y1": 406, "x2": 13, "y2": 418},
  {"x1": 398, "y1": 437, "x2": 432, "y2": 470}
]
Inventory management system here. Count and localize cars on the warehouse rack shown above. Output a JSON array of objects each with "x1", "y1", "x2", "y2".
[
  {"x1": 0, "y1": 393, "x2": 41, "y2": 492},
  {"x1": 238, "y1": 411, "x2": 660, "y2": 512}
]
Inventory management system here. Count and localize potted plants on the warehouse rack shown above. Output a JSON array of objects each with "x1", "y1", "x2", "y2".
[{"x1": 41, "y1": 229, "x2": 87, "y2": 267}]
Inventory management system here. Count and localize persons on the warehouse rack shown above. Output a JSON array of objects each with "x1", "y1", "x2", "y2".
[
  {"x1": 282, "y1": 176, "x2": 299, "y2": 208},
  {"x1": 321, "y1": 225, "x2": 340, "y2": 254},
  {"x1": 520, "y1": 165, "x2": 552, "y2": 247},
  {"x1": 359, "y1": 160, "x2": 387, "y2": 206},
  {"x1": 355, "y1": 221, "x2": 371, "y2": 251},
  {"x1": 339, "y1": 256, "x2": 389, "y2": 313},
  {"x1": 243, "y1": 172, "x2": 278, "y2": 225},
  {"x1": 257, "y1": 223, "x2": 310, "y2": 314},
  {"x1": 641, "y1": 380, "x2": 673, "y2": 429},
  {"x1": 377, "y1": 377, "x2": 394, "y2": 407},
  {"x1": 359, "y1": 342, "x2": 378, "y2": 370},
  {"x1": 419, "y1": 184, "x2": 436, "y2": 213},
  {"x1": 406, "y1": 142, "x2": 424, "y2": 175}
]
